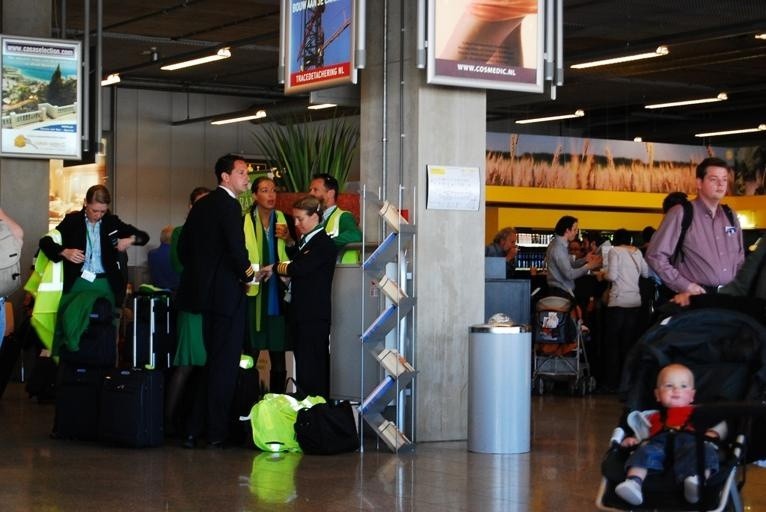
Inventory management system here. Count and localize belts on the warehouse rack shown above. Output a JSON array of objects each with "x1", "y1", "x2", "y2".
[{"x1": 698, "y1": 283, "x2": 726, "y2": 293}]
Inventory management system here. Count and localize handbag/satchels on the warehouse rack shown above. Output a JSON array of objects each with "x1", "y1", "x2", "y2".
[
  {"x1": 640, "y1": 277, "x2": 662, "y2": 309},
  {"x1": 237, "y1": 378, "x2": 327, "y2": 454},
  {"x1": 292, "y1": 399, "x2": 359, "y2": 457}
]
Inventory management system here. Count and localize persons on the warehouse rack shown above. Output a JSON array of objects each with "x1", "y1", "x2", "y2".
[
  {"x1": 238, "y1": 176, "x2": 292, "y2": 400},
  {"x1": 175, "y1": 153, "x2": 257, "y2": 451},
  {"x1": 597, "y1": 227, "x2": 644, "y2": 390},
  {"x1": 486, "y1": 227, "x2": 521, "y2": 279},
  {"x1": 307, "y1": 172, "x2": 364, "y2": 264},
  {"x1": 40, "y1": 185, "x2": 151, "y2": 364},
  {"x1": 535, "y1": 207, "x2": 605, "y2": 373},
  {"x1": 643, "y1": 156, "x2": 747, "y2": 306},
  {"x1": 568, "y1": 240, "x2": 584, "y2": 261},
  {"x1": 147, "y1": 225, "x2": 182, "y2": 291},
  {"x1": 580, "y1": 229, "x2": 606, "y2": 318},
  {"x1": 255, "y1": 193, "x2": 338, "y2": 399},
  {"x1": 614, "y1": 362, "x2": 729, "y2": 507},
  {"x1": 640, "y1": 225, "x2": 662, "y2": 284},
  {"x1": 0, "y1": 208, "x2": 25, "y2": 350},
  {"x1": 164, "y1": 186, "x2": 219, "y2": 437}
]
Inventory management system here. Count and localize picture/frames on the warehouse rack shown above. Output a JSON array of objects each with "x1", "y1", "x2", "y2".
[
  {"x1": 0, "y1": 35, "x2": 82, "y2": 161},
  {"x1": 279, "y1": 0, "x2": 367, "y2": 98}
]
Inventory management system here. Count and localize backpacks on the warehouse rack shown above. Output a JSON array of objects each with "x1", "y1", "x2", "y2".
[{"x1": 0, "y1": 218, "x2": 23, "y2": 297}]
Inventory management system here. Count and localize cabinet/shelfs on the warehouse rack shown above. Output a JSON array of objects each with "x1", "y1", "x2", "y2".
[{"x1": 359, "y1": 184, "x2": 415, "y2": 451}]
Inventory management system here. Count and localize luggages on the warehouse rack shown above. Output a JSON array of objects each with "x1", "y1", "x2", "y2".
[
  {"x1": 97, "y1": 292, "x2": 166, "y2": 450},
  {"x1": 50, "y1": 297, "x2": 117, "y2": 448}
]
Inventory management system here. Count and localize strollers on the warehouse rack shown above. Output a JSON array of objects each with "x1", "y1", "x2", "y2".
[
  {"x1": 594, "y1": 310, "x2": 765, "y2": 512},
  {"x1": 531, "y1": 279, "x2": 595, "y2": 396}
]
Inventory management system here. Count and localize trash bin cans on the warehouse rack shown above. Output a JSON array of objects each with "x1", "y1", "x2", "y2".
[{"x1": 468, "y1": 322, "x2": 533, "y2": 456}]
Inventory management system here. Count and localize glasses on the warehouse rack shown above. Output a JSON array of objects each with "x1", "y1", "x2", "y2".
[{"x1": 256, "y1": 186, "x2": 280, "y2": 194}]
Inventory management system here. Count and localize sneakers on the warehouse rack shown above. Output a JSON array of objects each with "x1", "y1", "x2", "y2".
[
  {"x1": 683, "y1": 477, "x2": 700, "y2": 505},
  {"x1": 614, "y1": 478, "x2": 643, "y2": 506}
]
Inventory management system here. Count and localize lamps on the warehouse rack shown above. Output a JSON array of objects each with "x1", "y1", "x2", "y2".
[
  {"x1": 632, "y1": 135, "x2": 644, "y2": 144},
  {"x1": 643, "y1": 92, "x2": 730, "y2": 111},
  {"x1": 307, "y1": 104, "x2": 336, "y2": 110},
  {"x1": 688, "y1": 123, "x2": 766, "y2": 138},
  {"x1": 754, "y1": 32, "x2": 766, "y2": 40},
  {"x1": 210, "y1": 107, "x2": 267, "y2": 126},
  {"x1": 512, "y1": 108, "x2": 586, "y2": 126},
  {"x1": 159, "y1": 47, "x2": 232, "y2": 71},
  {"x1": 100, "y1": 73, "x2": 120, "y2": 87},
  {"x1": 570, "y1": 44, "x2": 671, "y2": 72}
]
